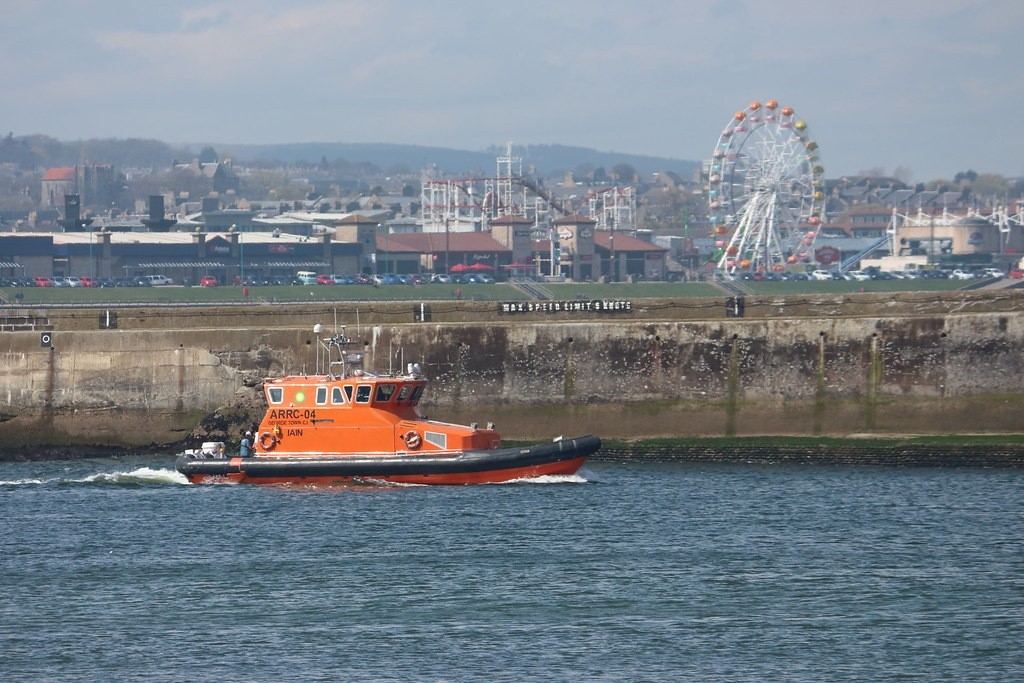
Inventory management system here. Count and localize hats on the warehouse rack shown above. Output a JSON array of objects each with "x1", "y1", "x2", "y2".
[{"x1": 246, "y1": 431, "x2": 251, "y2": 435}]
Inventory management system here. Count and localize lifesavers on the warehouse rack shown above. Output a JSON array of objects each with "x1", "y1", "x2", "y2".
[
  {"x1": 404, "y1": 431, "x2": 420, "y2": 448},
  {"x1": 259, "y1": 431, "x2": 276, "y2": 449}
]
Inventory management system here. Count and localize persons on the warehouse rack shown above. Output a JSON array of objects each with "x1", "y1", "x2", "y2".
[{"x1": 240, "y1": 431, "x2": 253, "y2": 457}]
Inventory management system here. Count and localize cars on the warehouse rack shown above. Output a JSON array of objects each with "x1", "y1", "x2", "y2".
[
  {"x1": 201, "y1": 276, "x2": 217, "y2": 287},
  {"x1": 0, "y1": 275, "x2": 174, "y2": 288},
  {"x1": 727, "y1": 268, "x2": 1024, "y2": 282},
  {"x1": 182, "y1": 277, "x2": 194, "y2": 286},
  {"x1": 233, "y1": 271, "x2": 496, "y2": 287}
]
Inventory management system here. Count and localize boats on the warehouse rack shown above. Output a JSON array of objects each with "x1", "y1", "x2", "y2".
[{"x1": 175, "y1": 324, "x2": 600, "y2": 484}]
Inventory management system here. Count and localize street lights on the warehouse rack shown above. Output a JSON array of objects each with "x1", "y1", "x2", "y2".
[
  {"x1": 82, "y1": 224, "x2": 93, "y2": 287},
  {"x1": 232, "y1": 224, "x2": 243, "y2": 286},
  {"x1": 378, "y1": 223, "x2": 388, "y2": 278}
]
